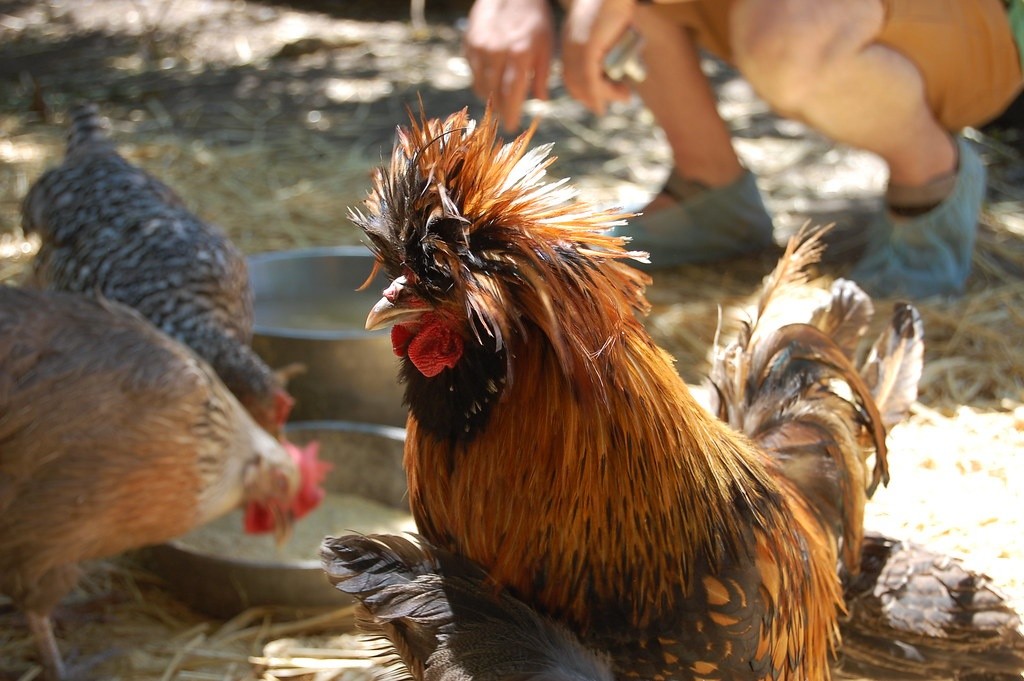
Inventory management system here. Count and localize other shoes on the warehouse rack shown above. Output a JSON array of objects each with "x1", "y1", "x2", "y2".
[
  {"x1": 849, "y1": 134, "x2": 984, "y2": 303},
  {"x1": 590, "y1": 161, "x2": 775, "y2": 274}
]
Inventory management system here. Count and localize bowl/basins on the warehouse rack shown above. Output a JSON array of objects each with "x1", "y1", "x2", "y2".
[
  {"x1": 149, "y1": 425, "x2": 408, "y2": 618},
  {"x1": 239, "y1": 250, "x2": 411, "y2": 422}
]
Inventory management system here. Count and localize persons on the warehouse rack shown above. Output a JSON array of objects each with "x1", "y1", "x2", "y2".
[{"x1": 462, "y1": 0, "x2": 1024, "y2": 304}]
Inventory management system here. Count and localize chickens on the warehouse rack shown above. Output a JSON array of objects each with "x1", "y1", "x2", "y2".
[
  {"x1": 318, "y1": 95, "x2": 925, "y2": 681},
  {"x1": 0, "y1": 96, "x2": 337, "y2": 681}
]
